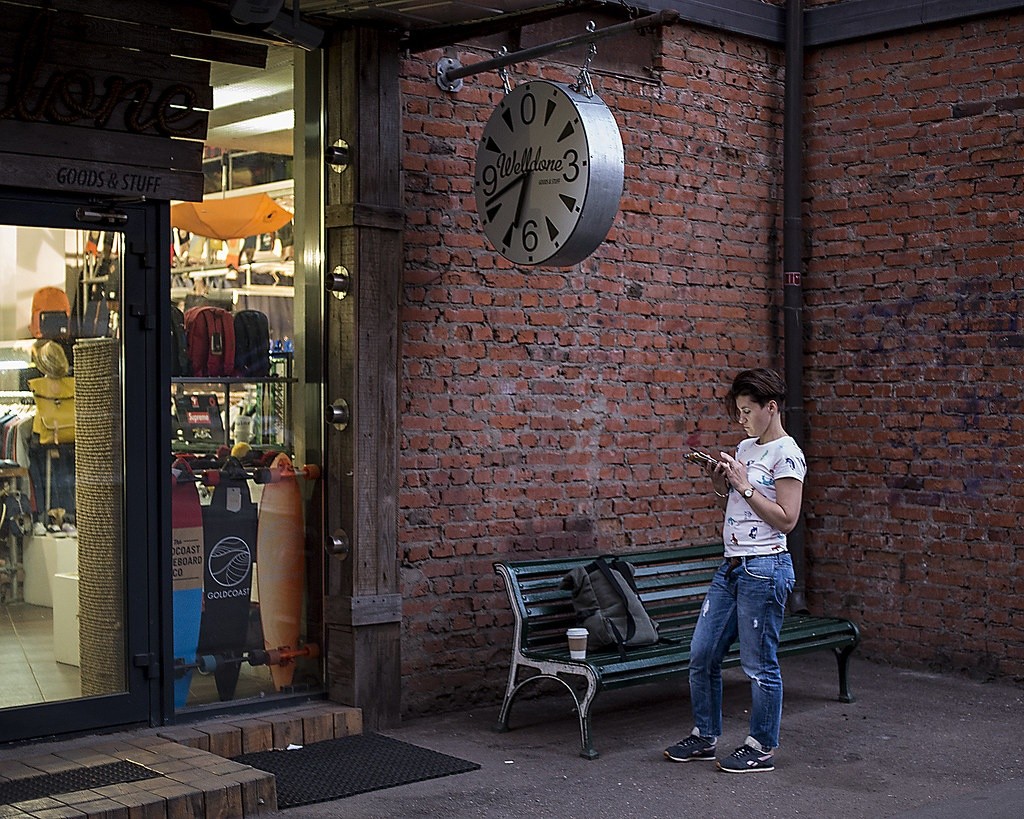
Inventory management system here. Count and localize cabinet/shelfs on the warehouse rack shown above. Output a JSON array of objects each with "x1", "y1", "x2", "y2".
[{"x1": 77, "y1": 145, "x2": 295, "y2": 472}]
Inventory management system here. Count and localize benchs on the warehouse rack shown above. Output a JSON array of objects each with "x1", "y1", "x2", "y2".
[{"x1": 491, "y1": 537, "x2": 859, "y2": 762}]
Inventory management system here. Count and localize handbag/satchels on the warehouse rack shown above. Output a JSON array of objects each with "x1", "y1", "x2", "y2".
[{"x1": 28, "y1": 377, "x2": 78, "y2": 444}]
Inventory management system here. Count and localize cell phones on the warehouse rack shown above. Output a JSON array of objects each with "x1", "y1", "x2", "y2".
[{"x1": 682, "y1": 452, "x2": 729, "y2": 479}]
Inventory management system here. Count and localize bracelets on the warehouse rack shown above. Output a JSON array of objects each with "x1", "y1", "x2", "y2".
[{"x1": 714, "y1": 489, "x2": 728, "y2": 498}]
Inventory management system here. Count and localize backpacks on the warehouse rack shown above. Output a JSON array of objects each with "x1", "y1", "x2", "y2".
[
  {"x1": 183, "y1": 306, "x2": 235, "y2": 378},
  {"x1": 232, "y1": 309, "x2": 271, "y2": 378},
  {"x1": 172, "y1": 306, "x2": 193, "y2": 376},
  {"x1": 560, "y1": 555, "x2": 659, "y2": 661}
]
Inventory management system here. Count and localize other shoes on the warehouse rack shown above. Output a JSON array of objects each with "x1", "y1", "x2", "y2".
[
  {"x1": 33, "y1": 523, "x2": 47, "y2": 537},
  {"x1": 63, "y1": 521, "x2": 78, "y2": 538},
  {"x1": 48, "y1": 524, "x2": 67, "y2": 537}
]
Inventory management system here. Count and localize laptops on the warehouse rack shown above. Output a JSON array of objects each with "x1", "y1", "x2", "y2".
[{"x1": 173, "y1": 393, "x2": 234, "y2": 445}]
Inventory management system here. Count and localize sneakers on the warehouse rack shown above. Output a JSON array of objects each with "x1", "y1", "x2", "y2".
[
  {"x1": 663, "y1": 727, "x2": 717, "y2": 761},
  {"x1": 717, "y1": 736, "x2": 774, "y2": 773}
]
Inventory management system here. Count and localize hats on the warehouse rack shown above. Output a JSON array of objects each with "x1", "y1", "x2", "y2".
[{"x1": 32, "y1": 339, "x2": 69, "y2": 378}]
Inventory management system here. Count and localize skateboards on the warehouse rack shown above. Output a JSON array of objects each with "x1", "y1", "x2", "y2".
[{"x1": 168, "y1": 450, "x2": 323, "y2": 711}]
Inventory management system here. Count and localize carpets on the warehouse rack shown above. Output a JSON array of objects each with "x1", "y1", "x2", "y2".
[{"x1": 229, "y1": 731, "x2": 483, "y2": 811}]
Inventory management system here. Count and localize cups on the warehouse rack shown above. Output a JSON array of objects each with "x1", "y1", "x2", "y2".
[
  {"x1": 233, "y1": 416, "x2": 253, "y2": 444},
  {"x1": 566, "y1": 628, "x2": 589, "y2": 659}
]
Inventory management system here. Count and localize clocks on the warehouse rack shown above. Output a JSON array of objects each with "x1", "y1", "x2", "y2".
[{"x1": 475, "y1": 80, "x2": 625, "y2": 269}]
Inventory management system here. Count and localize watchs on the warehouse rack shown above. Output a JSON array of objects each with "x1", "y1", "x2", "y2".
[{"x1": 743, "y1": 487, "x2": 755, "y2": 498}]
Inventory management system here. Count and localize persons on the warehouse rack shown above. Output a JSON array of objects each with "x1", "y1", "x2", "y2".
[{"x1": 662, "y1": 367, "x2": 806, "y2": 773}]
